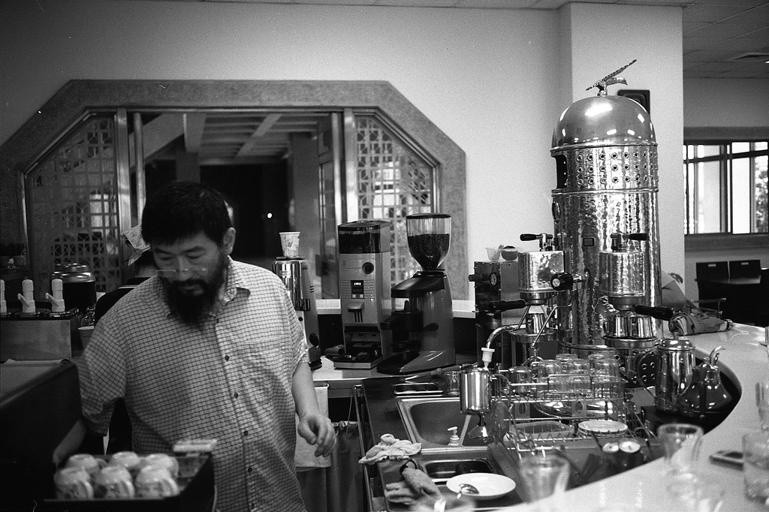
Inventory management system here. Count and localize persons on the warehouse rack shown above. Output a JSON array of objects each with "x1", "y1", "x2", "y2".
[
  {"x1": 87, "y1": 222, "x2": 160, "y2": 454},
  {"x1": 67, "y1": 178, "x2": 340, "y2": 512}
]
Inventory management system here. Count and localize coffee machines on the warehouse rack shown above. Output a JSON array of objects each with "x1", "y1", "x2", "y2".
[
  {"x1": 271, "y1": 257, "x2": 323, "y2": 371},
  {"x1": 331, "y1": 218, "x2": 393, "y2": 370},
  {"x1": 468, "y1": 260, "x2": 529, "y2": 370},
  {"x1": 378, "y1": 213, "x2": 457, "y2": 375}
]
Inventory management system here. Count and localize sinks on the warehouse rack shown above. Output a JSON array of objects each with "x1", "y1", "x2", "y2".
[{"x1": 395, "y1": 397, "x2": 508, "y2": 454}]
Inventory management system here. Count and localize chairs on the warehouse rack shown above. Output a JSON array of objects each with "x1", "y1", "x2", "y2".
[{"x1": 695, "y1": 256, "x2": 769, "y2": 329}]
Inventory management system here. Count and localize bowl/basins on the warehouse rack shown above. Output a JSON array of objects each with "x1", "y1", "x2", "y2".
[{"x1": 445, "y1": 472, "x2": 517, "y2": 501}]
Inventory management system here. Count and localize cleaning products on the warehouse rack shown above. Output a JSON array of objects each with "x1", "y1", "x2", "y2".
[{"x1": 447, "y1": 425, "x2": 459, "y2": 446}]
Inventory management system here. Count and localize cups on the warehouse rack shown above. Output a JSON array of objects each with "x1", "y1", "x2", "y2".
[
  {"x1": 77, "y1": 325, "x2": 95, "y2": 351},
  {"x1": 532, "y1": 346, "x2": 623, "y2": 413},
  {"x1": 51, "y1": 449, "x2": 181, "y2": 498},
  {"x1": 656, "y1": 379, "x2": 769, "y2": 512},
  {"x1": 441, "y1": 369, "x2": 461, "y2": 396},
  {"x1": 653, "y1": 339, "x2": 696, "y2": 412},
  {"x1": 518, "y1": 455, "x2": 571, "y2": 511},
  {"x1": 276, "y1": 231, "x2": 301, "y2": 259},
  {"x1": 486, "y1": 247, "x2": 502, "y2": 263}
]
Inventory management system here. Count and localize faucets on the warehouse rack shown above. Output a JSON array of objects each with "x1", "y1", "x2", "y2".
[{"x1": 480, "y1": 326, "x2": 538, "y2": 373}]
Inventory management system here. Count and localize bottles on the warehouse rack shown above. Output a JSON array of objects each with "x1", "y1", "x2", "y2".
[{"x1": 679, "y1": 358, "x2": 732, "y2": 420}]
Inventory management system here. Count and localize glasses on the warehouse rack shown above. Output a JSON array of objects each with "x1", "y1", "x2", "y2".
[{"x1": 151, "y1": 251, "x2": 219, "y2": 278}]
